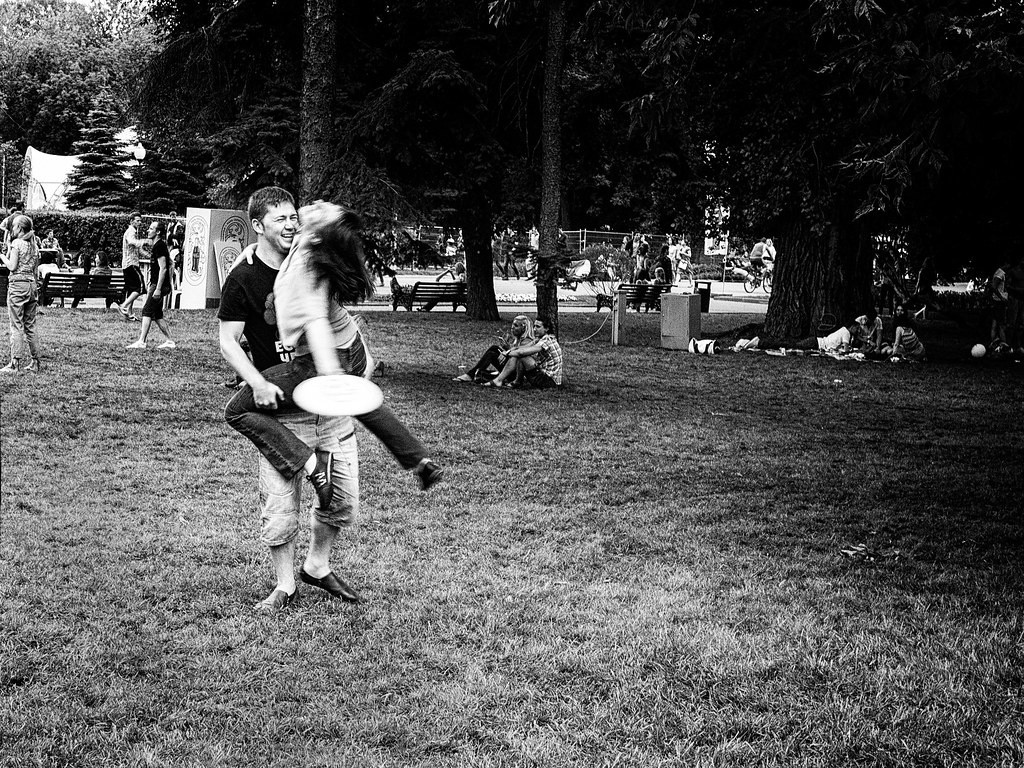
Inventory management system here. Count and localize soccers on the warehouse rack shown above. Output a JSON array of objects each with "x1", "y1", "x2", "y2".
[{"x1": 971, "y1": 343, "x2": 986, "y2": 357}]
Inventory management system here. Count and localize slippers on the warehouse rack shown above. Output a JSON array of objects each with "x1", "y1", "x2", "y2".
[
  {"x1": 480, "y1": 379, "x2": 501, "y2": 389},
  {"x1": 507, "y1": 380, "x2": 523, "y2": 387}
]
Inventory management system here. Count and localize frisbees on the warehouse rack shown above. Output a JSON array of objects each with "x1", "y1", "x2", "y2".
[{"x1": 292, "y1": 372, "x2": 386, "y2": 418}]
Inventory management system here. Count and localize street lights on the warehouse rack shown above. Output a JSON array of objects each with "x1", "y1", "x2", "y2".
[{"x1": 135, "y1": 142, "x2": 147, "y2": 214}]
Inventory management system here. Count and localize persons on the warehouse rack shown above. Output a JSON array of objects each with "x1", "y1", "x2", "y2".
[
  {"x1": 166, "y1": 210, "x2": 185, "y2": 283},
  {"x1": 126, "y1": 221, "x2": 175, "y2": 348},
  {"x1": 37, "y1": 252, "x2": 112, "y2": 286},
  {"x1": 42, "y1": 228, "x2": 71, "y2": 269},
  {"x1": 854, "y1": 303, "x2": 927, "y2": 362},
  {"x1": 621, "y1": 231, "x2": 694, "y2": 312},
  {"x1": 741, "y1": 320, "x2": 860, "y2": 352},
  {"x1": 445, "y1": 238, "x2": 457, "y2": 256},
  {"x1": 912, "y1": 257, "x2": 938, "y2": 319},
  {"x1": 217, "y1": 187, "x2": 360, "y2": 608},
  {"x1": 118, "y1": 213, "x2": 153, "y2": 322},
  {"x1": 878, "y1": 262, "x2": 898, "y2": 315},
  {"x1": 224, "y1": 202, "x2": 444, "y2": 513},
  {"x1": 726, "y1": 237, "x2": 776, "y2": 280},
  {"x1": 417, "y1": 262, "x2": 466, "y2": 312},
  {"x1": 0, "y1": 203, "x2": 24, "y2": 243},
  {"x1": 452, "y1": 315, "x2": 536, "y2": 382},
  {"x1": 490, "y1": 227, "x2": 539, "y2": 280},
  {"x1": 479, "y1": 317, "x2": 563, "y2": 389},
  {"x1": 990, "y1": 259, "x2": 1012, "y2": 341},
  {"x1": 558, "y1": 225, "x2": 567, "y2": 248},
  {"x1": 0, "y1": 215, "x2": 41, "y2": 374}
]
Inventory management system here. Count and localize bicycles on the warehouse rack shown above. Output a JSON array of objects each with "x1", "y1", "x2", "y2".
[{"x1": 744, "y1": 263, "x2": 773, "y2": 294}]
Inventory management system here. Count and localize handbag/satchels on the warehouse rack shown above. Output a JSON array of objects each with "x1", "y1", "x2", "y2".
[
  {"x1": 678, "y1": 260, "x2": 688, "y2": 270},
  {"x1": 688, "y1": 337, "x2": 721, "y2": 355}
]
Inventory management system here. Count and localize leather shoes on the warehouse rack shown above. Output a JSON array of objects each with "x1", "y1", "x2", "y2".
[
  {"x1": 258, "y1": 586, "x2": 298, "y2": 611},
  {"x1": 299, "y1": 566, "x2": 369, "y2": 605}
]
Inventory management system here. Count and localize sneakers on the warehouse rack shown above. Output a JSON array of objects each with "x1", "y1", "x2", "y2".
[
  {"x1": 305, "y1": 450, "x2": 333, "y2": 513},
  {"x1": 418, "y1": 460, "x2": 444, "y2": 491}
]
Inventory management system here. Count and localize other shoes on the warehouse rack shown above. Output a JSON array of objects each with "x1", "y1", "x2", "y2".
[
  {"x1": 23, "y1": 357, "x2": 39, "y2": 373},
  {"x1": 452, "y1": 373, "x2": 476, "y2": 381},
  {"x1": 743, "y1": 337, "x2": 760, "y2": 350},
  {"x1": 417, "y1": 307, "x2": 429, "y2": 312},
  {"x1": 127, "y1": 314, "x2": 141, "y2": 323},
  {"x1": 157, "y1": 340, "x2": 175, "y2": 348},
  {"x1": 118, "y1": 304, "x2": 130, "y2": 318},
  {"x1": 672, "y1": 284, "x2": 678, "y2": 287},
  {"x1": 1, "y1": 361, "x2": 21, "y2": 373},
  {"x1": 688, "y1": 284, "x2": 693, "y2": 288},
  {"x1": 126, "y1": 341, "x2": 147, "y2": 348}
]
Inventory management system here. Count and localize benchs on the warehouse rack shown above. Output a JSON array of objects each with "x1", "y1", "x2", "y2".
[
  {"x1": 390, "y1": 280, "x2": 469, "y2": 313},
  {"x1": 38, "y1": 272, "x2": 127, "y2": 308},
  {"x1": 596, "y1": 284, "x2": 672, "y2": 314}
]
